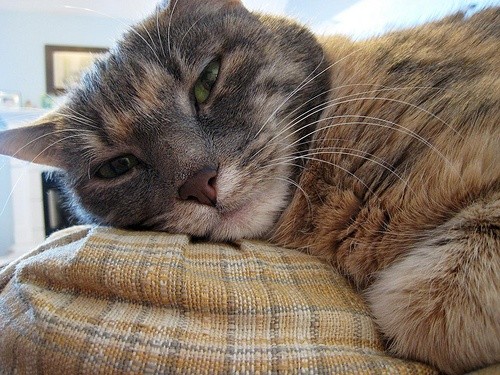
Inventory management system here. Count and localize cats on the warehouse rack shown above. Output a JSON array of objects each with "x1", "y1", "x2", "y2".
[{"x1": 0, "y1": 0, "x2": 500, "y2": 374}]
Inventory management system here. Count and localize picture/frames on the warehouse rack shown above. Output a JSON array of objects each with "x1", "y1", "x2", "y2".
[{"x1": 45, "y1": 42, "x2": 111, "y2": 95}]
